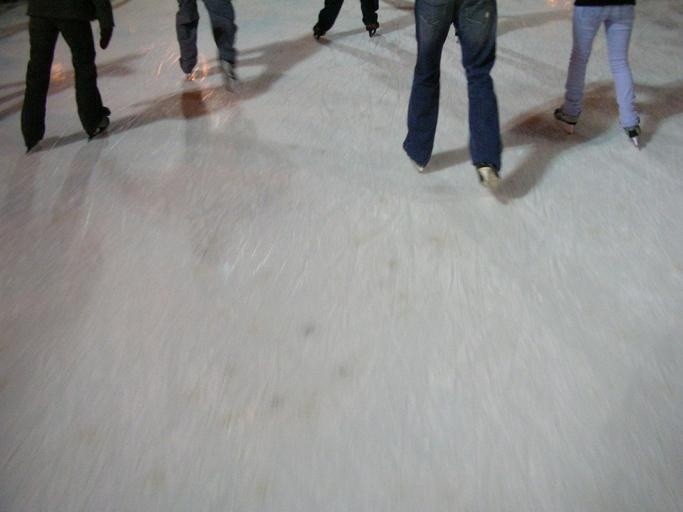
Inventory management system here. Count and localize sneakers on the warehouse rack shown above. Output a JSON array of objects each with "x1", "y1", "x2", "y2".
[
  {"x1": 554, "y1": 109, "x2": 577, "y2": 125},
  {"x1": 624, "y1": 117, "x2": 641, "y2": 137}
]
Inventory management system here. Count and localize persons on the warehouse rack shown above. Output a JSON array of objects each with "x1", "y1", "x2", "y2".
[
  {"x1": 312, "y1": 1, "x2": 380, "y2": 36},
  {"x1": 555, "y1": 0, "x2": 641, "y2": 137},
  {"x1": 403, "y1": 0, "x2": 502, "y2": 184},
  {"x1": 20, "y1": 1, "x2": 114, "y2": 147},
  {"x1": 176, "y1": 0, "x2": 239, "y2": 80}
]
